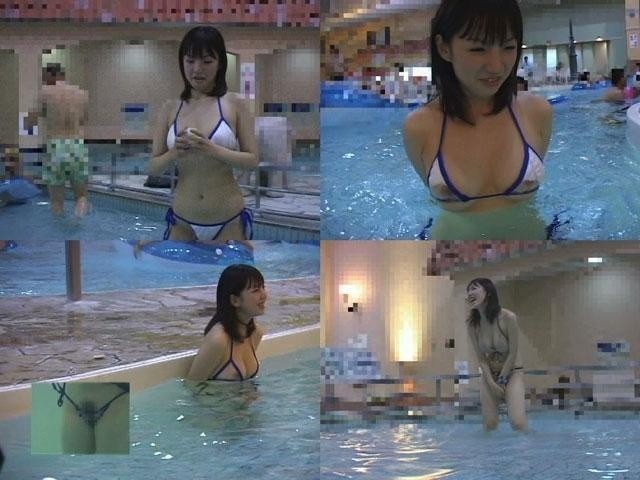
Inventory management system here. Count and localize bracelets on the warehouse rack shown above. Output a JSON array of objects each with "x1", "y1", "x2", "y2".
[{"x1": 495, "y1": 377, "x2": 510, "y2": 386}]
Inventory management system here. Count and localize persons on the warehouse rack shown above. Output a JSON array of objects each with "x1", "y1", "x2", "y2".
[
  {"x1": 403, "y1": 1, "x2": 554, "y2": 239},
  {"x1": 607, "y1": 69, "x2": 625, "y2": 100},
  {"x1": 147, "y1": 26, "x2": 259, "y2": 244},
  {"x1": 524, "y1": 55, "x2": 535, "y2": 80},
  {"x1": 51, "y1": 384, "x2": 129, "y2": 455},
  {"x1": 186, "y1": 263, "x2": 266, "y2": 412},
  {"x1": 465, "y1": 277, "x2": 527, "y2": 433},
  {"x1": 28, "y1": 63, "x2": 89, "y2": 219}
]
